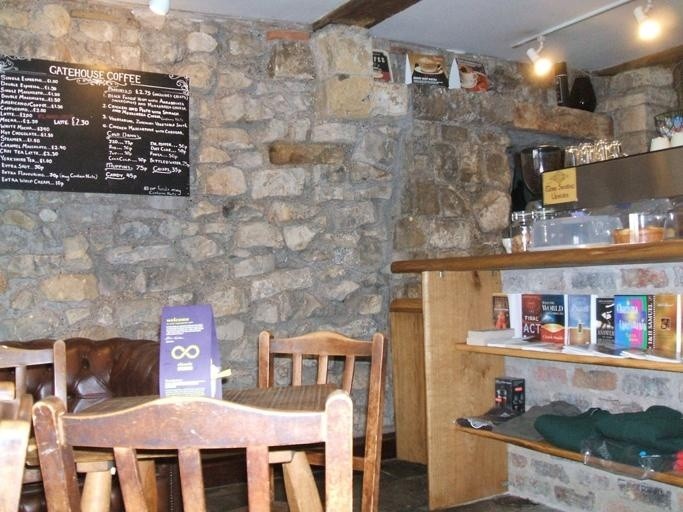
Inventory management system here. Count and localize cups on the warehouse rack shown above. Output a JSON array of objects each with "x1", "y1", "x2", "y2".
[
  {"x1": 419, "y1": 61, "x2": 443, "y2": 72},
  {"x1": 460, "y1": 70, "x2": 478, "y2": 84},
  {"x1": 564, "y1": 138, "x2": 623, "y2": 167},
  {"x1": 374, "y1": 67, "x2": 383, "y2": 74}
]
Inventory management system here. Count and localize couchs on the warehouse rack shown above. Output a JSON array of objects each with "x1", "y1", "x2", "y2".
[{"x1": 0, "y1": 335, "x2": 214, "y2": 512}]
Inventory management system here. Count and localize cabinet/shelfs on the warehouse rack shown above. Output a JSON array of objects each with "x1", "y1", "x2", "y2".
[{"x1": 388, "y1": 240, "x2": 682, "y2": 511}]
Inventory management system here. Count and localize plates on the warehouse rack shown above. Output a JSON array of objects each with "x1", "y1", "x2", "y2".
[
  {"x1": 373, "y1": 73, "x2": 384, "y2": 78},
  {"x1": 460, "y1": 77, "x2": 478, "y2": 89},
  {"x1": 415, "y1": 66, "x2": 444, "y2": 76}
]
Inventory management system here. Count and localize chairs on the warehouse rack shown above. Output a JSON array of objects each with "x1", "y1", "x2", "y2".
[
  {"x1": 0, "y1": 338, "x2": 72, "y2": 423},
  {"x1": 256, "y1": 326, "x2": 387, "y2": 512},
  {"x1": 1, "y1": 381, "x2": 35, "y2": 512}
]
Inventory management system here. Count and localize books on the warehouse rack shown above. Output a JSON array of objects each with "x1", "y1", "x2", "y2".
[{"x1": 460, "y1": 290, "x2": 683, "y2": 363}]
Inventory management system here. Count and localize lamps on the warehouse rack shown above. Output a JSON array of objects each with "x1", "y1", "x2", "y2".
[
  {"x1": 525, "y1": 36, "x2": 553, "y2": 76},
  {"x1": 627, "y1": 0, "x2": 659, "y2": 43}
]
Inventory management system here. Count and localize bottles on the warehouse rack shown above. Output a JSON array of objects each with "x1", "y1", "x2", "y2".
[
  {"x1": 509, "y1": 207, "x2": 555, "y2": 253},
  {"x1": 555, "y1": 62, "x2": 570, "y2": 107},
  {"x1": 570, "y1": 76, "x2": 597, "y2": 112}
]
